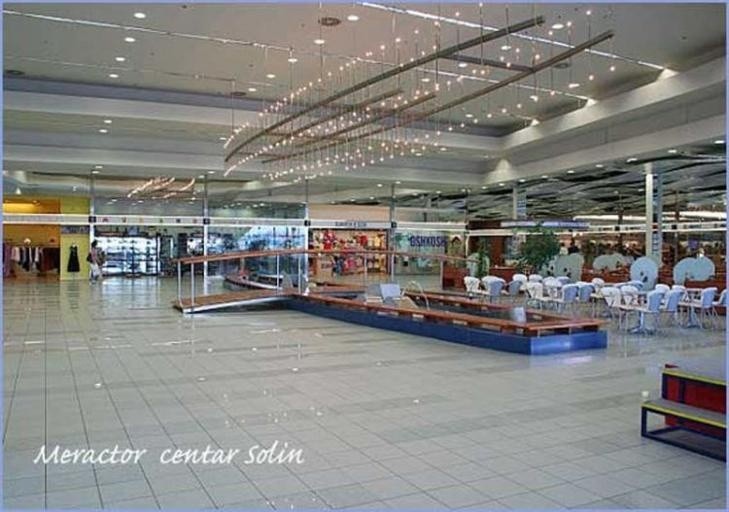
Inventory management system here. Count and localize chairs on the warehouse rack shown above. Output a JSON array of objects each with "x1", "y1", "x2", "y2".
[{"x1": 464, "y1": 273, "x2": 729, "y2": 336}]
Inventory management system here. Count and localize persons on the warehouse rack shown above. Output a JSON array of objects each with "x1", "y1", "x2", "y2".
[
  {"x1": 559, "y1": 242, "x2": 569, "y2": 255},
  {"x1": 567, "y1": 241, "x2": 580, "y2": 253},
  {"x1": 90, "y1": 239, "x2": 100, "y2": 284}
]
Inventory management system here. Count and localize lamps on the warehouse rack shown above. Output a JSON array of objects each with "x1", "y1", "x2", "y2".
[{"x1": 223, "y1": 5, "x2": 615, "y2": 182}]
